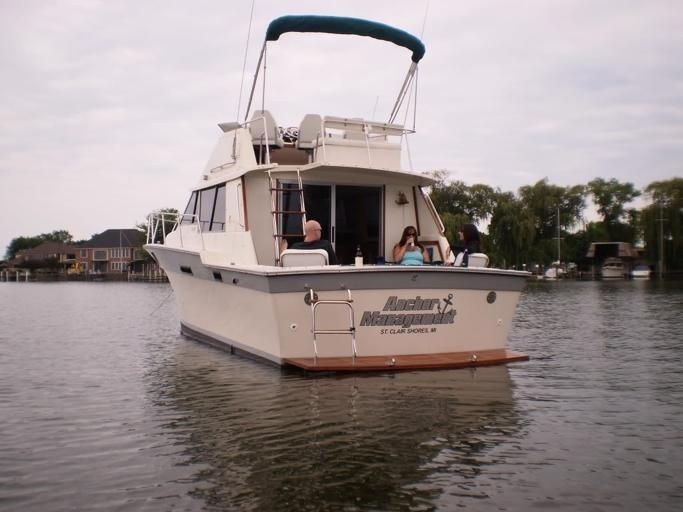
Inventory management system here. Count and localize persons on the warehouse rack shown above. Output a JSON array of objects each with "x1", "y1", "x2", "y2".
[
  {"x1": 280, "y1": 220, "x2": 338, "y2": 267},
  {"x1": 444, "y1": 224, "x2": 480, "y2": 266},
  {"x1": 393, "y1": 225, "x2": 430, "y2": 265}
]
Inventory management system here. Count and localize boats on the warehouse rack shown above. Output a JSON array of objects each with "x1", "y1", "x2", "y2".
[
  {"x1": 631, "y1": 264, "x2": 651, "y2": 278},
  {"x1": 600, "y1": 256, "x2": 624, "y2": 280},
  {"x1": 165, "y1": 336, "x2": 514, "y2": 512},
  {"x1": 132, "y1": 9, "x2": 539, "y2": 381}
]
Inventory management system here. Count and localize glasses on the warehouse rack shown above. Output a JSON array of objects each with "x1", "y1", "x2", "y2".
[{"x1": 406, "y1": 233, "x2": 416, "y2": 237}]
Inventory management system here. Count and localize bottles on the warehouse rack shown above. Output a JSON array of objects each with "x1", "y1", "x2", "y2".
[
  {"x1": 354, "y1": 244, "x2": 363, "y2": 268},
  {"x1": 408, "y1": 235, "x2": 414, "y2": 248}
]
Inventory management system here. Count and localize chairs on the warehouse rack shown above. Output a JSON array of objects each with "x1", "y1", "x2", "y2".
[
  {"x1": 293, "y1": 113, "x2": 325, "y2": 164},
  {"x1": 248, "y1": 109, "x2": 284, "y2": 163},
  {"x1": 278, "y1": 248, "x2": 331, "y2": 269},
  {"x1": 453, "y1": 251, "x2": 491, "y2": 268}
]
